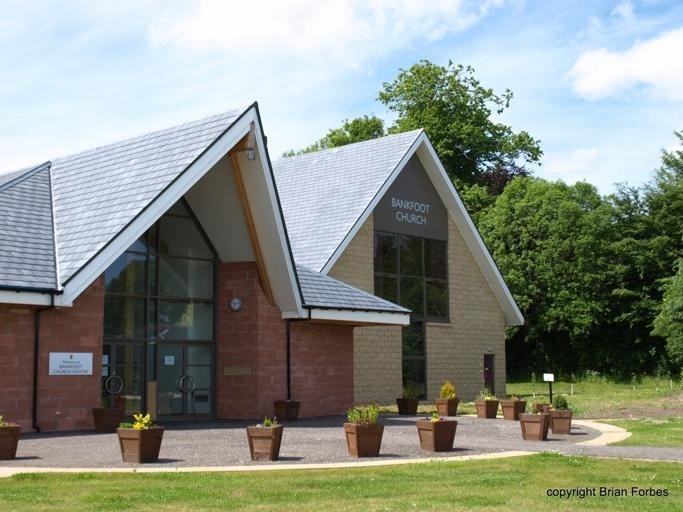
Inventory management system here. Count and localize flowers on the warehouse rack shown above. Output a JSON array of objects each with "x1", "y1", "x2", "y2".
[
  {"x1": 439, "y1": 381, "x2": 456, "y2": 400},
  {"x1": 424, "y1": 412, "x2": 444, "y2": 423},
  {"x1": 119, "y1": 413, "x2": 155, "y2": 431}
]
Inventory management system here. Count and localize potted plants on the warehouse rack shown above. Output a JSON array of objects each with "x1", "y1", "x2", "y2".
[
  {"x1": 92, "y1": 398, "x2": 122, "y2": 433},
  {"x1": 395, "y1": 386, "x2": 418, "y2": 415},
  {"x1": 473, "y1": 388, "x2": 572, "y2": 441},
  {"x1": 0, "y1": 415, "x2": 21, "y2": 460},
  {"x1": 342, "y1": 400, "x2": 383, "y2": 458},
  {"x1": 246, "y1": 416, "x2": 282, "y2": 462}
]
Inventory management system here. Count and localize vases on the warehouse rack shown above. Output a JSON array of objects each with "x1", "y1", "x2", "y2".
[
  {"x1": 435, "y1": 399, "x2": 458, "y2": 416},
  {"x1": 416, "y1": 419, "x2": 457, "y2": 452},
  {"x1": 274, "y1": 400, "x2": 299, "y2": 420},
  {"x1": 115, "y1": 427, "x2": 165, "y2": 463}
]
